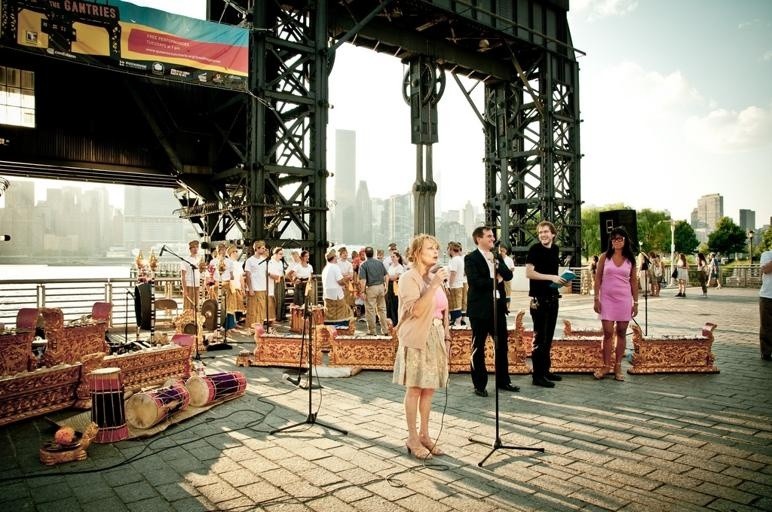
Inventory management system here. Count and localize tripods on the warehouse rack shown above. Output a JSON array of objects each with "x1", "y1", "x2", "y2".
[
  {"x1": 110, "y1": 295, "x2": 139, "y2": 350},
  {"x1": 615, "y1": 315, "x2": 646, "y2": 338},
  {"x1": 220, "y1": 293, "x2": 243, "y2": 344},
  {"x1": 268, "y1": 297, "x2": 348, "y2": 434},
  {"x1": 468, "y1": 251, "x2": 544, "y2": 466},
  {"x1": 189, "y1": 269, "x2": 215, "y2": 366}
]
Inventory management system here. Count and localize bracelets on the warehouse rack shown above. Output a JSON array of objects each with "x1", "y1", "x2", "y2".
[
  {"x1": 634, "y1": 302, "x2": 638, "y2": 304},
  {"x1": 444, "y1": 338, "x2": 453, "y2": 342}
]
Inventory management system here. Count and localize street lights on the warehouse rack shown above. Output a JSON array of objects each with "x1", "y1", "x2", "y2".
[
  {"x1": 747, "y1": 230, "x2": 754, "y2": 275},
  {"x1": 638, "y1": 239, "x2": 643, "y2": 251},
  {"x1": 657, "y1": 211, "x2": 688, "y2": 289}
]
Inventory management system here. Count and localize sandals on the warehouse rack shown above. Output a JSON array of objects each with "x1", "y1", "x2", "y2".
[
  {"x1": 593, "y1": 364, "x2": 611, "y2": 379},
  {"x1": 613, "y1": 363, "x2": 626, "y2": 381}
]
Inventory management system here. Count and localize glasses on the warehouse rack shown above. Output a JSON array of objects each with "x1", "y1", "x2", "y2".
[{"x1": 611, "y1": 237, "x2": 625, "y2": 242}]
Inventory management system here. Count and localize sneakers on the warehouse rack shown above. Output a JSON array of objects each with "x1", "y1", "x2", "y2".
[{"x1": 716, "y1": 284, "x2": 722, "y2": 289}]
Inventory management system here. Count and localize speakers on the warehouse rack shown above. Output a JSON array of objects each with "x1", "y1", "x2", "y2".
[{"x1": 599, "y1": 210, "x2": 639, "y2": 256}]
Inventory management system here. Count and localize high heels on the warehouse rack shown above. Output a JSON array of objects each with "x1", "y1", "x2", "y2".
[
  {"x1": 420, "y1": 436, "x2": 444, "y2": 456},
  {"x1": 407, "y1": 439, "x2": 432, "y2": 461}
]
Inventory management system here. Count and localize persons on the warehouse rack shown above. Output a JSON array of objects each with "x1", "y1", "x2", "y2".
[
  {"x1": 524, "y1": 219, "x2": 573, "y2": 388},
  {"x1": 391, "y1": 232, "x2": 453, "y2": 460},
  {"x1": 590, "y1": 227, "x2": 640, "y2": 382},
  {"x1": 444, "y1": 242, "x2": 467, "y2": 325},
  {"x1": 180, "y1": 240, "x2": 314, "y2": 332},
  {"x1": 498, "y1": 243, "x2": 515, "y2": 308},
  {"x1": 590, "y1": 250, "x2": 721, "y2": 299},
  {"x1": 463, "y1": 226, "x2": 521, "y2": 398},
  {"x1": 758, "y1": 250, "x2": 771, "y2": 362},
  {"x1": 321, "y1": 243, "x2": 411, "y2": 339}
]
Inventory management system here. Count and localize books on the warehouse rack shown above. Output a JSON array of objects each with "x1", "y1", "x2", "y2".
[{"x1": 549, "y1": 269, "x2": 577, "y2": 289}]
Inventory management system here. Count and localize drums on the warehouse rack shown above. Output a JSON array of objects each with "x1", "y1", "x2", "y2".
[
  {"x1": 86, "y1": 368, "x2": 129, "y2": 444},
  {"x1": 185, "y1": 370, "x2": 246, "y2": 405},
  {"x1": 126, "y1": 383, "x2": 190, "y2": 430}
]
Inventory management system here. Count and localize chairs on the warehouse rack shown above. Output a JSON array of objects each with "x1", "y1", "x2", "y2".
[
  {"x1": 0, "y1": 301, "x2": 121, "y2": 377},
  {"x1": 152, "y1": 298, "x2": 179, "y2": 333}
]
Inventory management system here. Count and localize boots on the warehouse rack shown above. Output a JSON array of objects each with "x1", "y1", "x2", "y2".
[{"x1": 675, "y1": 293, "x2": 687, "y2": 297}]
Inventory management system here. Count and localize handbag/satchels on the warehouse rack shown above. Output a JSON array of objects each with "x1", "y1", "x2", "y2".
[
  {"x1": 639, "y1": 252, "x2": 651, "y2": 270},
  {"x1": 671, "y1": 268, "x2": 678, "y2": 278}
]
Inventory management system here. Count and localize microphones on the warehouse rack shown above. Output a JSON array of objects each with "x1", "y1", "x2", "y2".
[
  {"x1": 488, "y1": 240, "x2": 500, "y2": 251},
  {"x1": 436, "y1": 261, "x2": 451, "y2": 292},
  {"x1": 127, "y1": 289, "x2": 136, "y2": 299},
  {"x1": 283, "y1": 374, "x2": 301, "y2": 386},
  {"x1": 283, "y1": 238, "x2": 295, "y2": 248},
  {"x1": 159, "y1": 245, "x2": 166, "y2": 256}
]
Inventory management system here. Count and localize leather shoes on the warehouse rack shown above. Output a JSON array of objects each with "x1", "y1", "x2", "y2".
[
  {"x1": 497, "y1": 383, "x2": 521, "y2": 393},
  {"x1": 532, "y1": 376, "x2": 556, "y2": 388},
  {"x1": 546, "y1": 371, "x2": 563, "y2": 382},
  {"x1": 474, "y1": 387, "x2": 489, "y2": 398}
]
